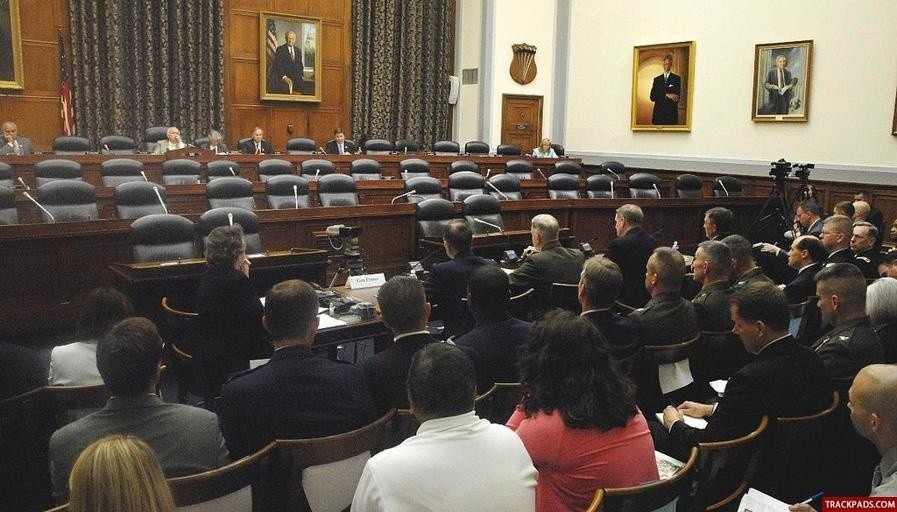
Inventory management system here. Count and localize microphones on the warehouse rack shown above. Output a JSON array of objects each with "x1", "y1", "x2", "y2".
[
  {"x1": 484, "y1": 169, "x2": 490, "y2": 180},
  {"x1": 486, "y1": 181, "x2": 509, "y2": 200},
  {"x1": 140, "y1": 170, "x2": 148, "y2": 182},
  {"x1": 23, "y1": 191, "x2": 56, "y2": 223},
  {"x1": 314, "y1": 168, "x2": 321, "y2": 181},
  {"x1": 610, "y1": 180, "x2": 613, "y2": 198},
  {"x1": 474, "y1": 218, "x2": 502, "y2": 231},
  {"x1": 405, "y1": 169, "x2": 408, "y2": 180},
  {"x1": 537, "y1": 168, "x2": 548, "y2": 180},
  {"x1": 227, "y1": 213, "x2": 233, "y2": 226},
  {"x1": 607, "y1": 168, "x2": 620, "y2": 180},
  {"x1": 152, "y1": 186, "x2": 168, "y2": 215},
  {"x1": 293, "y1": 184, "x2": 299, "y2": 209},
  {"x1": 392, "y1": 189, "x2": 417, "y2": 204},
  {"x1": 230, "y1": 166, "x2": 236, "y2": 176},
  {"x1": 255, "y1": 148, "x2": 259, "y2": 156},
  {"x1": 718, "y1": 179, "x2": 728, "y2": 197},
  {"x1": 404, "y1": 147, "x2": 407, "y2": 157},
  {"x1": 320, "y1": 146, "x2": 327, "y2": 155},
  {"x1": 653, "y1": 183, "x2": 661, "y2": 198},
  {"x1": 104, "y1": 144, "x2": 110, "y2": 151},
  {"x1": 18, "y1": 177, "x2": 30, "y2": 190}
]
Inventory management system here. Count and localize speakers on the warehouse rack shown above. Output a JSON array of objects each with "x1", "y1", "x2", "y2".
[{"x1": 445, "y1": 74, "x2": 461, "y2": 106}]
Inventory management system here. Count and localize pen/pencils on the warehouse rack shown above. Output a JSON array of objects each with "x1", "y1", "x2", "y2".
[
  {"x1": 774, "y1": 242, "x2": 777, "y2": 246},
  {"x1": 798, "y1": 491, "x2": 825, "y2": 507},
  {"x1": 669, "y1": 398, "x2": 679, "y2": 411},
  {"x1": 244, "y1": 259, "x2": 251, "y2": 266}
]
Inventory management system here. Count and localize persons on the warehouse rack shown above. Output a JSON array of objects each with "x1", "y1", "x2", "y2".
[
  {"x1": 532, "y1": 138, "x2": 559, "y2": 159},
  {"x1": 324, "y1": 127, "x2": 358, "y2": 155},
  {"x1": 276, "y1": 31, "x2": 304, "y2": 94},
  {"x1": 763, "y1": 55, "x2": 791, "y2": 114},
  {"x1": 650, "y1": 55, "x2": 681, "y2": 125},
  {"x1": 151, "y1": 127, "x2": 186, "y2": 155},
  {"x1": 0, "y1": 121, "x2": 41, "y2": 155},
  {"x1": 242, "y1": 127, "x2": 274, "y2": 154},
  {"x1": 0, "y1": 189, "x2": 897, "y2": 512},
  {"x1": 205, "y1": 130, "x2": 228, "y2": 154}
]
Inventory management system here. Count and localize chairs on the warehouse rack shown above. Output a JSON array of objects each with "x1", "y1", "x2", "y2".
[{"x1": 1, "y1": 127, "x2": 897, "y2": 512}]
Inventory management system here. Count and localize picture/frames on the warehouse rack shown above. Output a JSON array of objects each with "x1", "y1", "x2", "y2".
[
  {"x1": 751, "y1": 40, "x2": 813, "y2": 123},
  {"x1": 0, "y1": 0, "x2": 25, "y2": 91},
  {"x1": 260, "y1": 10, "x2": 322, "y2": 103},
  {"x1": 631, "y1": 41, "x2": 696, "y2": 133}
]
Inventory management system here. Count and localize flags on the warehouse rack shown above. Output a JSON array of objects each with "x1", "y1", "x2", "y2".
[
  {"x1": 266, "y1": 20, "x2": 277, "y2": 64},
  {"x1": 58, "y1": 35, "x2": 76, "y2": 137}
]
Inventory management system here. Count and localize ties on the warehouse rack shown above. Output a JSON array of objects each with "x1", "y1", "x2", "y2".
[
  {"x1": 12, "y1": 143, "x2": 18, "y2": 154},
  {"x1": 290, "y1": 48, "x2": 294, "y2": 62},
  {"x1": 256, "y1": 144, "x2": 259, "y2": 149},
  {"x1": 340, "y1": 144, "x2": 343, "y2": 154},
  {"x1": 779, "y1": 70, "x2": 784, "y2": 89},
  {"x1": 664, "y1": 73, "x2": 669, "y2": 83}
]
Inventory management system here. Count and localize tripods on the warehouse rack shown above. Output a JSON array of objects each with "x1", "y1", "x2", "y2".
[{"x1": 740, "y1": 183, "x2": 819, "y2": 250}]
